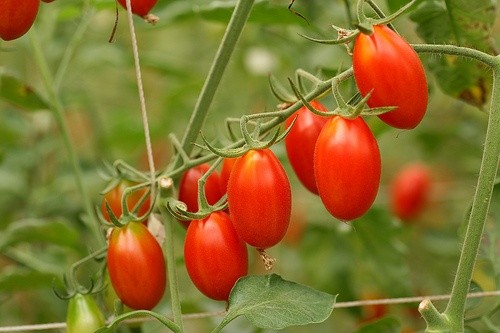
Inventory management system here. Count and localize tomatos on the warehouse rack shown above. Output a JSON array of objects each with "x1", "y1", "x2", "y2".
[
  {"x1": 0, "y1": 0, "x2": 56, "y2": 41},
  {"x1": 117, "y1": 0, "x2": 158, "y2": 17},
  {"x1": 66, "y1": 19, "x2": 433, "y2": 333}
]
